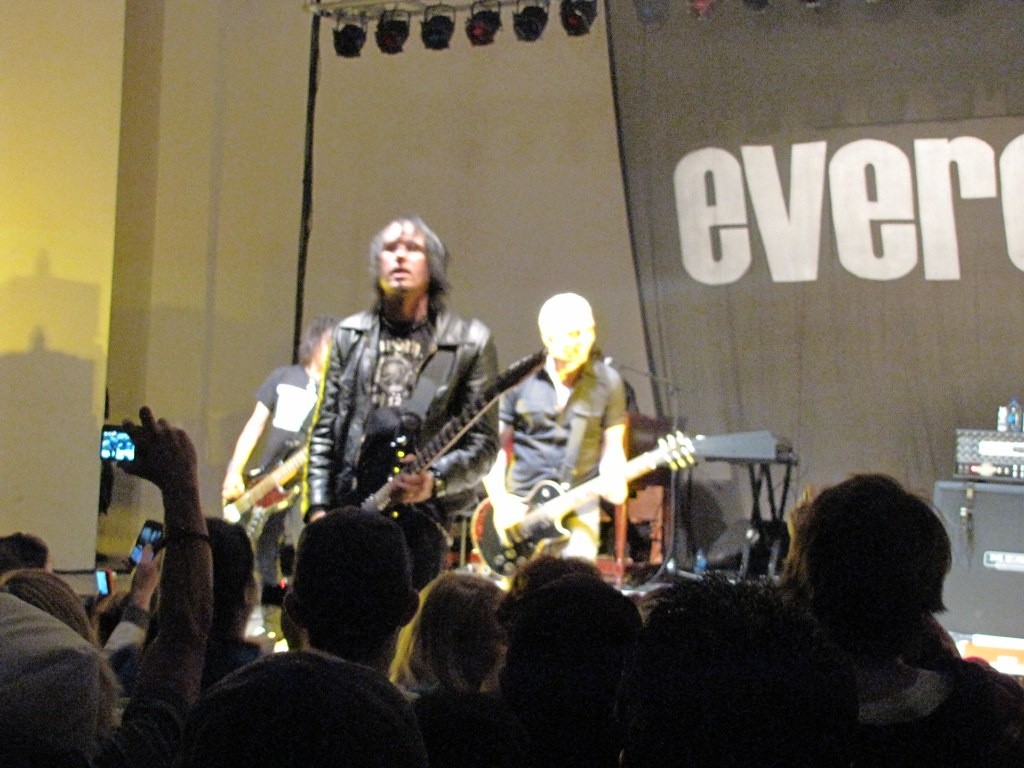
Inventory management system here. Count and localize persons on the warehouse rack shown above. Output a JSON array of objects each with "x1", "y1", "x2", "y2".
[
  {"x1": 0, "y1": 405, "x2": 1024, "y2": 768},
  {"x1": 302, "y1": 216, "x2": 500, "y2": 592},
  {"x1": 217, "y1": 315, "x2": 339, "y2": 586},
  {"x1": 480, "y1": 293, "x2": 628, "y2": 562}
]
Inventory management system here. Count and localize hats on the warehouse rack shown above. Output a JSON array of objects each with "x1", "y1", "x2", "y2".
[{"x1": 294, "y1": 506, "x2": 409, "y2": 628}]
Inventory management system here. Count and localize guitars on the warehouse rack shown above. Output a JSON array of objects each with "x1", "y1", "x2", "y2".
[
  {"x1": 339, "y1": 349, "x2": 546, "y2": 586},
  {"x1": 469, "y1": 428, "x2": 697, "y2": 579},
  {"x1": 222, "y1": 438, "x2": 315, "y2": 535}
]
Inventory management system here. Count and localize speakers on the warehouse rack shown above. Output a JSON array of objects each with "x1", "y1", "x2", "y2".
[{"x1": 926, "y1": 477, "x2": 1024, "y2": 642}]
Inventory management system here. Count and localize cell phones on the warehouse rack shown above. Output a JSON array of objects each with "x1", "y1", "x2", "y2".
[
  {"x1": 99, "y1": 423, "x2": 136, "y2": 461},
  {"x1": 95, "y1": 568, "x2": 109, "y2": 598},
  {"x1": 128, "y1": 520, "x2": 164, "y2": 566}
]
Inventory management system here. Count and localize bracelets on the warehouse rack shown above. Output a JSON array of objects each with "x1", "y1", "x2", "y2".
[
  {"x1": 433, "y1": 471, "x2": 446, "y2": 498},
  {"x1": 161, "y1": 531, "x2": 210, "y2": 547}
]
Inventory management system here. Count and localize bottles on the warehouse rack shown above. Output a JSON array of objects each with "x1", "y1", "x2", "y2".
[
  {"x1": 694, "y1": 548, "x2": 706, "y2": 573},
  {"x1": 1007, "y1": 396, "x2": 1022, "y2": 431}
]
